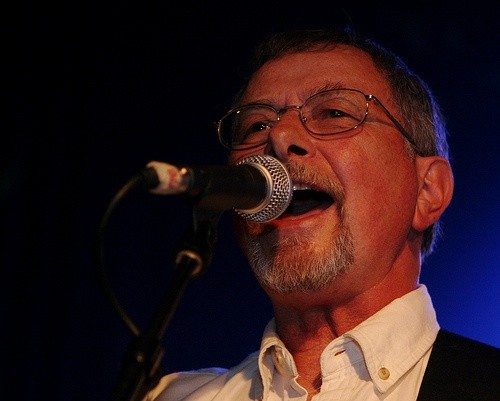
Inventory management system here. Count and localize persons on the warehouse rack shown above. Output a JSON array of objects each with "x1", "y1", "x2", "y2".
[{"x1": 135, "y1": 26, "x2": 499, "y2": 400}]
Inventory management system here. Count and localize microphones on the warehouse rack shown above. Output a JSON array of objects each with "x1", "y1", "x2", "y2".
[{"x1": 146, "y1": 153, "x2": 294, "y2": 222}]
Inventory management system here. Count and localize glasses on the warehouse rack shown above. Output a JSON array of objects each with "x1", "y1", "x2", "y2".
[{"x1": 216, "y1": 87, "x2": 417, "y2": 150}]
